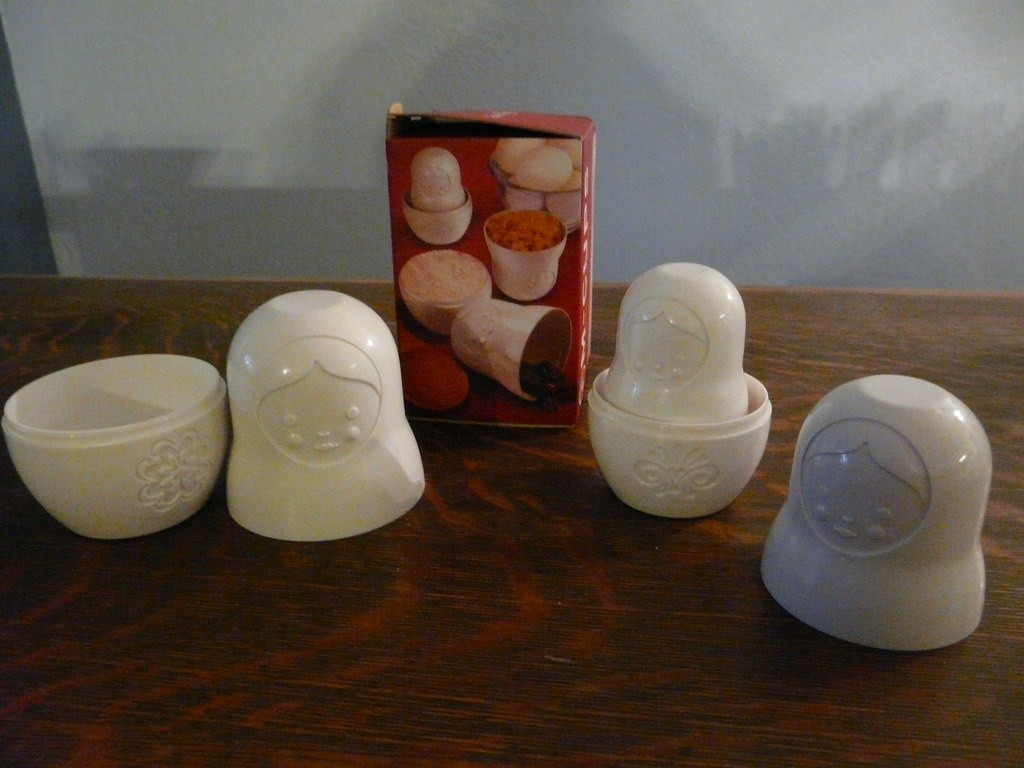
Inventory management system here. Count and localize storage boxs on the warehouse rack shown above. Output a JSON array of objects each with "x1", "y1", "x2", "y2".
[{"x1": 382, "y1": 100, "x2": 595, "y2": 430}]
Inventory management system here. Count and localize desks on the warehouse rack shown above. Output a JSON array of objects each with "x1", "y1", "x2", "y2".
[{"x1": 0, "y1": 273, "x2": 1024, "y2": 767}]
